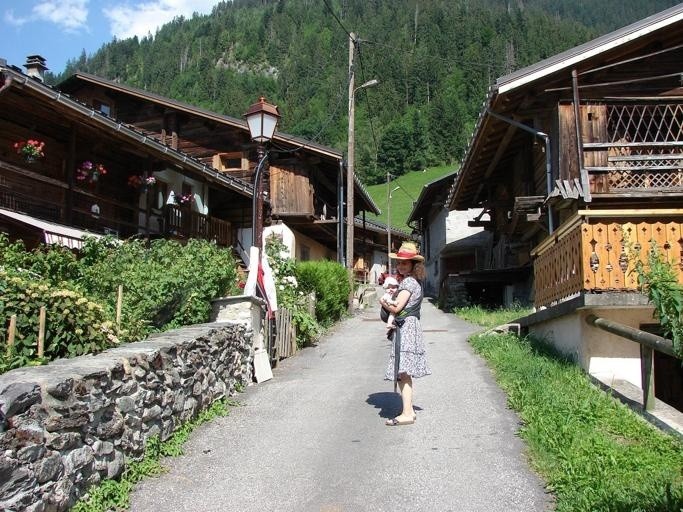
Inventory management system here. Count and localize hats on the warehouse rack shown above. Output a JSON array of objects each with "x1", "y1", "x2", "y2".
[
  {"x1": 382, "y1": 276, "x2": 401, "y2": 289},
  {"x1": 388, "y1": 242, "x2": 426, "y2": 262}
]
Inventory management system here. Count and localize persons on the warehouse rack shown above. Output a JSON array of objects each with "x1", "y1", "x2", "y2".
[
  {"x1": 379, "y1": 240, "x2": 427, "y2": 425},
  {"x1": 379, "y1": 275, "x2": 400, "y2": 332}
]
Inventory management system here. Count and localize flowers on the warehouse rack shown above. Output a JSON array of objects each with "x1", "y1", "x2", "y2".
[
  {"x1": 9, "y1": 138, "x2": 48, "y2": 160},
  {"x1": 172, "y1": 191, "x2": 195, "y2": 206},
  {"x1": 127, "y1": 175, "x2": 156, "y2": 189},
  {"x1": 76, "y1": 161, "x2": 107, "y2": 190}
]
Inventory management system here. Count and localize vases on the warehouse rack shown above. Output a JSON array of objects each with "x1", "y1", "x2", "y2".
[{"x1": 25, "y1": 153, "x2": 36, "y2": 163}]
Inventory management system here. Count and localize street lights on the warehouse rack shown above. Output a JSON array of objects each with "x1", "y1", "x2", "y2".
[
  {"x1": 387, "y1": 186, "x2": 401, "y2": 275},
  {"x1": 245, "y1": 97, "x2": 282, "y2": 248},
  {"x1": 346, "y1": 78, "x2": 378, "y2": 311}
]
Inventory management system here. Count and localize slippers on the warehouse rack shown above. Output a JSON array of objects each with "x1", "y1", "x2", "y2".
[{"x1": 384, "y1": 418, "x2": 415, "y2": 426}]
